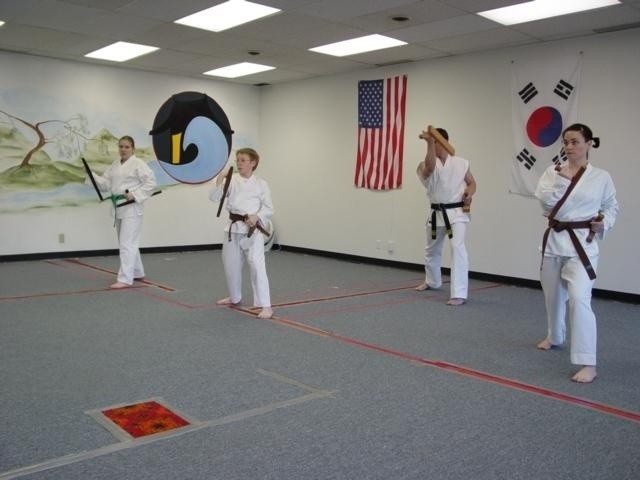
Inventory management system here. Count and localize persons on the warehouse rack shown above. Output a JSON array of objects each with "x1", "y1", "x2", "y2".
[
  {"x1": 413, "y1": 128, "x2": 476, "y2": 307},
  {"x1": 83, "y1": 135, "x2": 157, "y2": 288},
  {"x1": 208, "y1": 147, "x2": 274, "y2": 319},
  {"x1": 534, "y1": 123, "x2": 618, "y2": 384}
]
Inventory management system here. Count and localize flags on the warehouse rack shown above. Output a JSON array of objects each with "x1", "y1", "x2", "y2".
[
  {"x1": 354, "y1": 73, "x2": 408, "y2": 191},
  {"x1": 508, "y1": 50, "x2": 583, "y2": 197}
]
